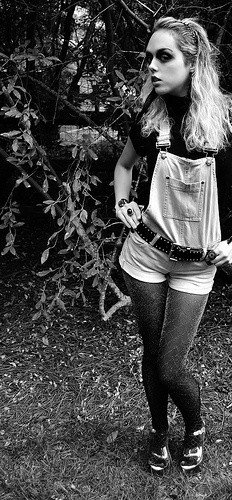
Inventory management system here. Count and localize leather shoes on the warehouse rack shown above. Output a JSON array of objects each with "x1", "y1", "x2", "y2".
[{"x1": 137, "y1": 219, "x2": 213, "y2": 264}]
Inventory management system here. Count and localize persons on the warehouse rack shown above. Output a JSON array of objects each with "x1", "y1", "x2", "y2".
[{"x1": 110, "y1": 13, "x2": 232, "y2": 476}]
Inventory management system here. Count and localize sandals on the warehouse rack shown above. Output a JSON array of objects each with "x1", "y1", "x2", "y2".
[
  {"x1": 149, "y1": 427, "x2": 170, "y2": 470},
  {"x1": 179, "y1": 424, "x2": 204, "y2": 470}
]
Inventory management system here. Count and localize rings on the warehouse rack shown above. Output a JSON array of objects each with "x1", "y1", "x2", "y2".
[
  {"x1": 207, "y1": 250, "x2": 217, "y2": 260},
  {"x1": 126, "y1": 207, "x2": 134, "y2": 217}
]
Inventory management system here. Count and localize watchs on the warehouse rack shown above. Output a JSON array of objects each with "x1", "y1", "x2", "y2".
[{"x1": 116, "y1": 198, "x2": 130, "y2": 211}]
